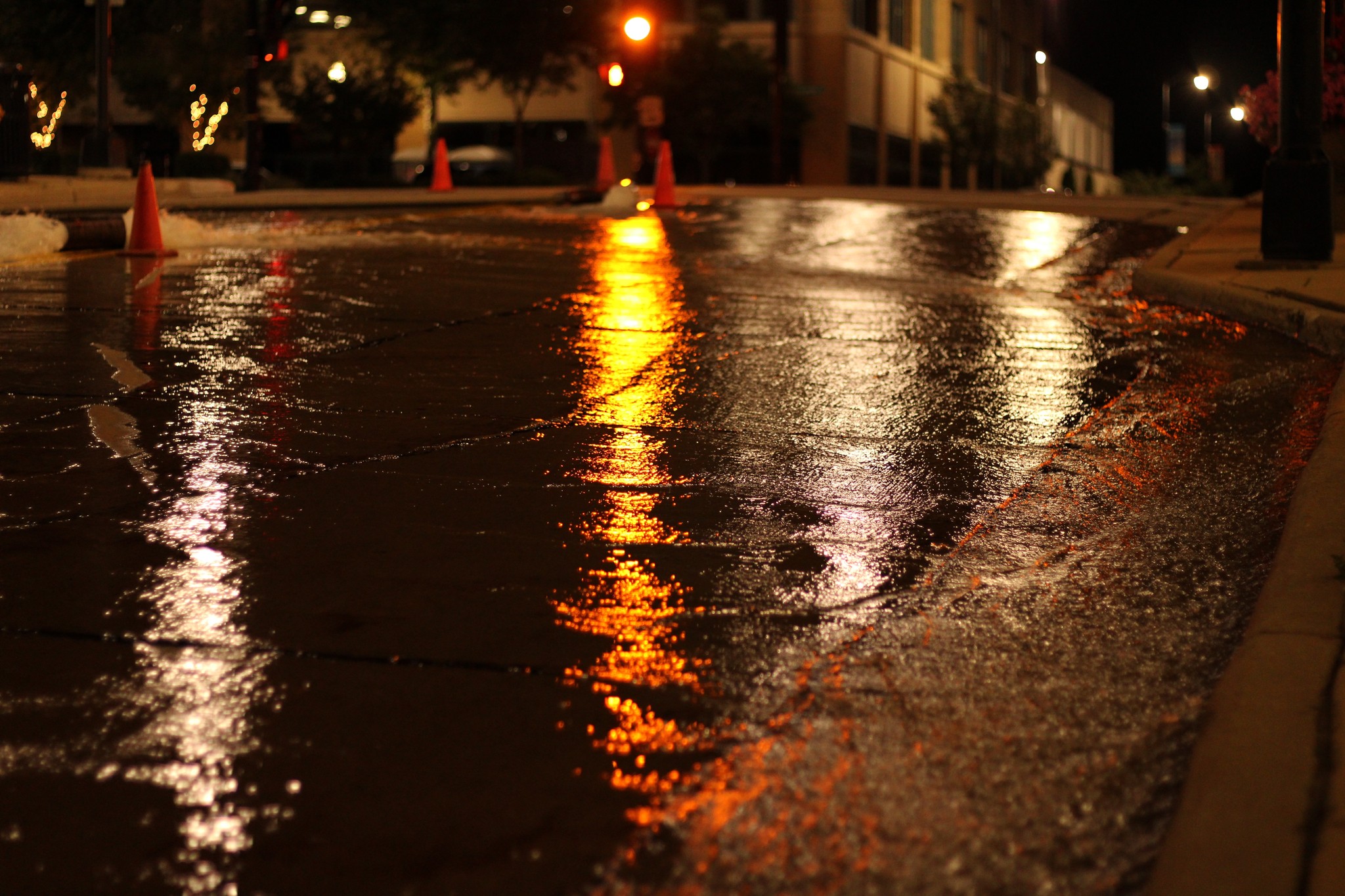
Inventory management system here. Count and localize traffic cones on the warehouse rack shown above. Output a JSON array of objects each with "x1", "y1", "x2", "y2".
[
  {"x1": 593, "y1": 135, "x2": 621, "y2": 197},
  {"x1": 424, "y1": 137, "x2": 454, "y2": 195},
  {"x1": 646, "y1": 140, "x2": 679, "y2": 209},
  {"x1": 117, "y1": 160, "x2": 179, "y2": 260}
]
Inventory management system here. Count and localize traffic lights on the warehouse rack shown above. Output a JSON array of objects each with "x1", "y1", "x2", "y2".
[{"x1": 621, "y1": 6, "x2": 662, "y2": 93}]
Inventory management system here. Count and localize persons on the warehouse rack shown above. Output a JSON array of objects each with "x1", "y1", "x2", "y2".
[{"x1": 589, "y1": 90, "x2": 670, "y2": 187}]
